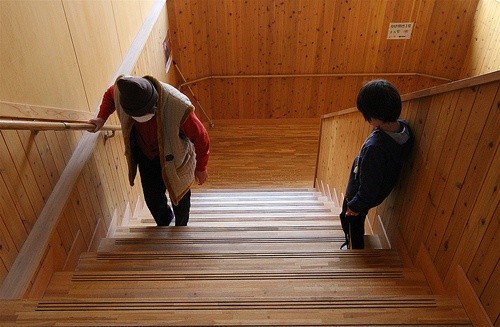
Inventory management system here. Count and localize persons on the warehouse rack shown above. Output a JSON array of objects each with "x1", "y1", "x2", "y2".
[
  {"x1": 339, "y1": 80, "x2": 412, "y2": 250},
  {"x1": 87, "y1": 75, "x2": 211, "y2": 226}
]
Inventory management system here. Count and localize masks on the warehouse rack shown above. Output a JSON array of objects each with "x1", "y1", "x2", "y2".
[{"x1": 131, "y1": 113, "x2": 154, "y2": 123}]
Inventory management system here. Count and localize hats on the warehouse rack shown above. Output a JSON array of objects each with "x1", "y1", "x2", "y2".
[{"x1": 116, "y1": 76, "x2": 157, "y2": 116}]
